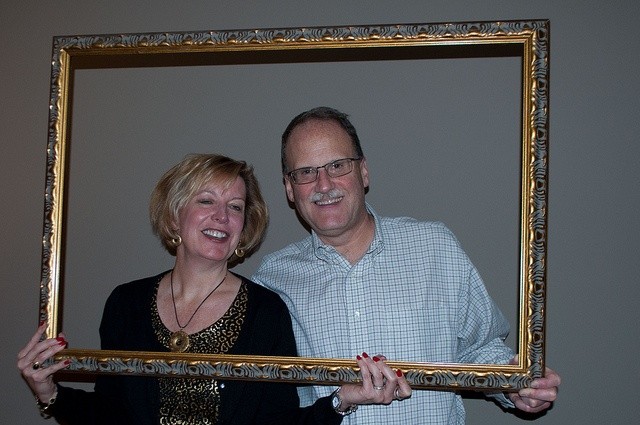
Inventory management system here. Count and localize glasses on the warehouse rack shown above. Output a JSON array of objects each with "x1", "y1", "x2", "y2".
[{"x1": 288, "y1": 156, "x2": 360, "y2": 185}]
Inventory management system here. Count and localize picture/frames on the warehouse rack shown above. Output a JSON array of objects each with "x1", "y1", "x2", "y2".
[{"x1": 33, "y1": 16, "x2": 551, "y2": 395}]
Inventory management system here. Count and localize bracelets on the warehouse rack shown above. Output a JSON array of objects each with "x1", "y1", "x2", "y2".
[{"x1": 35, "y1": 383, "x2": 57, "y2": 412}]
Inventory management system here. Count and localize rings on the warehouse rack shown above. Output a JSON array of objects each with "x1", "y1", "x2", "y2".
[
  {"x1": 31, "y1": 359, "x2": 43, "y2": 370},
  {"x1": 373, "y1": 383, "x2": 386, "y2": 390},
  {"x1": 394, "y1": 386, "x2": 403, "y2": 401}
]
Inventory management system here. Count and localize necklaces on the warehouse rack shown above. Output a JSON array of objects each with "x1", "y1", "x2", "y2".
[{"x1": 170, "y1": 270, "x2": 227, "y2": 352}]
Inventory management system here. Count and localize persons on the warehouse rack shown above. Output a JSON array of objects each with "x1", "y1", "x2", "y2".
[
  {"x1": 14, "y1": 151, "x2": 413, "y2": 425},
  {"x1": 252, "y1": 107, "x2": 561, "y2": 425}
]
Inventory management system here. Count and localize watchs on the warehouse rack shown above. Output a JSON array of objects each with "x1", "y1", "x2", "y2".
[{"x1": 332, "y1": 387, "x2": 358, "y2": 415}]
